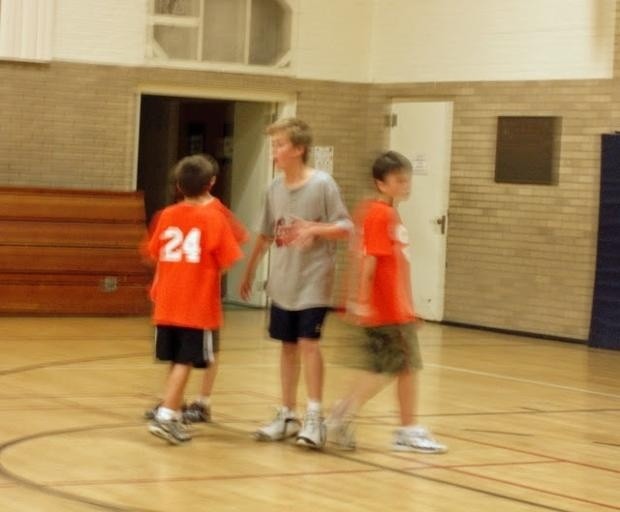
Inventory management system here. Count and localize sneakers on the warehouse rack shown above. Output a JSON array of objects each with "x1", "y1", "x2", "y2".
[
  {"x1": 256, "y1": 406, "x2": 303, "y2": 442},
  {"x1": 321, "y1": 409, "x2": 357, "y2": 453},
  {"x1": 183, "y1": 402, "x2": 211, "y2": 423},
  {"x1": 295, "y1": 404, "x2": 326, "y2": 449},
  {"x1": 149, "y1": 413, "x2": 193, "y2": 446},
  {"x1": 393, "y1": 425, "x2": 447, "y2": 454}
]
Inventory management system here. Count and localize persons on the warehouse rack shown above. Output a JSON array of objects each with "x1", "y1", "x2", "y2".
[
  {"x1": 323, "y1": 150, "x2": 450, "y2": 456},
  {"x1": 238, "y1": 116, "x2": 357, "y2": 451}
]
